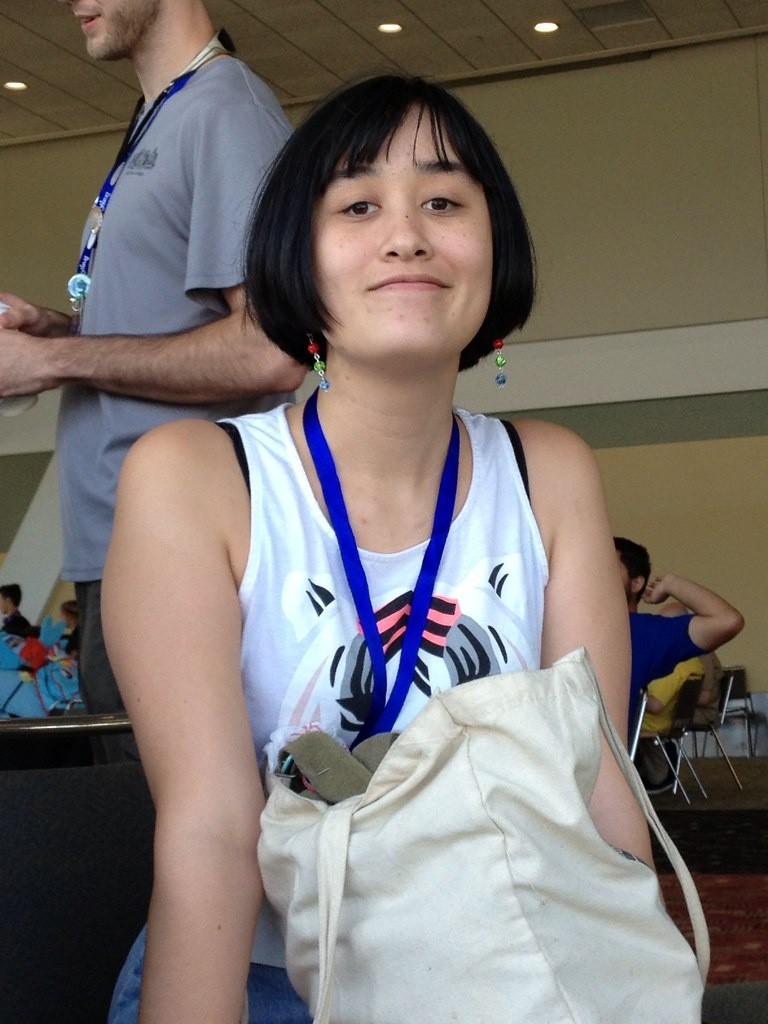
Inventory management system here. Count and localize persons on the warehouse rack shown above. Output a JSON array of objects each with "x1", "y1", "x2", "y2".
[
  {"x1": 100, "y1": 71, "x2": 663, "y2": 1024},
  {"x1": 1, "y1": 0, "x2": 310, "y2": 762},
  {"x1": 0, "y1": 532, "x2": 745, "y2": 793}
]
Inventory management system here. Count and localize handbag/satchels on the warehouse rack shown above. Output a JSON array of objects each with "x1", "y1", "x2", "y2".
[{"x1": 256, "y1": 646, "x2": 710, "y2": 1024}]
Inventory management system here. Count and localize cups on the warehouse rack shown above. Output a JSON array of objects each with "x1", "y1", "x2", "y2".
[{"x1": 0, "y1": 303, "x2": 40, "y2": 416}]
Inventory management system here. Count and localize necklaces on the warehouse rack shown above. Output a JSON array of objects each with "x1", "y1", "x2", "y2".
[{"x1": 66, "y1": 54, "x2": 192, "y2": 310}]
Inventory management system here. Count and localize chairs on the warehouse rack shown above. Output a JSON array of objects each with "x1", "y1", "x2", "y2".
[
  {"x1": 0, "y1": 698, "x2": 157, "y2": 1024},
  {"x1": 628, "y1": 667, "x2": 755, "y2": 805}
]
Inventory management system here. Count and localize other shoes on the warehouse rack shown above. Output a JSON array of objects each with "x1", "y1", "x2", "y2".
[{"x1": 640, "y1": 769, "x2": 675, "y2": 794}]
